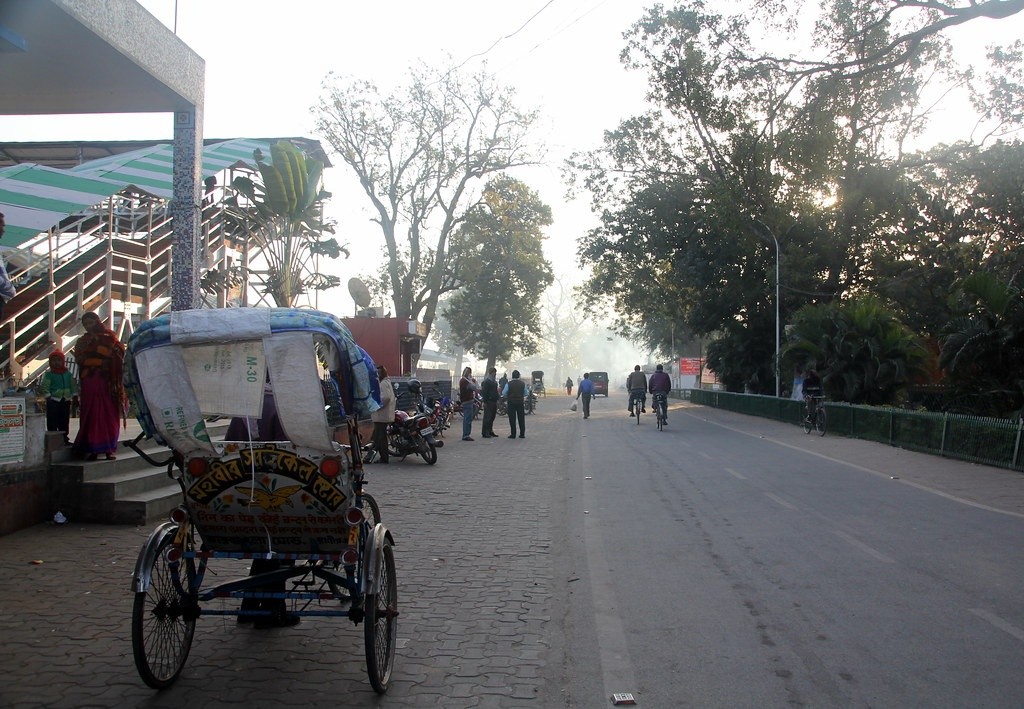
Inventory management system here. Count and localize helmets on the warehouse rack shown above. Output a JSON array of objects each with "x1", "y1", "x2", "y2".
[{"x1": 407, "y1": 378, "x2": 422, "y2": 394}]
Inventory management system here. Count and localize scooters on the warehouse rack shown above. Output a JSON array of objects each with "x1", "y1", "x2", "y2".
[{"x1": 414, "y1": 381, "x2": 454, "y2": 439}]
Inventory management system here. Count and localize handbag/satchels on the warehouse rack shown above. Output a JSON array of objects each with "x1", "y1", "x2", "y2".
[{"x1": 570, "y1": 399, "x2": 577, "y2": 411}]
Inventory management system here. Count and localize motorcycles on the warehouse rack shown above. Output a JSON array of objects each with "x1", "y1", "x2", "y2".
[{"x1": 387, "y1": 382, "x2": 444, "y2": 466}]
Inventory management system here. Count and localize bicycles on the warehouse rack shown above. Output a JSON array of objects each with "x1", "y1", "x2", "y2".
[
  {"x1": 451, "y1": 387, "x2": 539, "y2": 419},
  {"x1": 802, "y1": 394, "x2": 828, "y2": 437},
  {"x1": 653, "y1": 393, "x2": 667, "y2": 431},
  {"x1": 628, "y1": 390, "x2": 645, "y2": 425}
]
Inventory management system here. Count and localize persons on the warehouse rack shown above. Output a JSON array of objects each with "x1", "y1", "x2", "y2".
[
  {"x1": 649, "y1": 364, "x2": 671, "y2": 425},
  {"x1": 802, "y1": 369, "x2": 825, "y2": 428},
  {"x1": 576, "y1": 373, "x2": 595, "y2": 418},
  {"x1": 502, "y1": 370, "x2": 528, "y2": 438},
  {"x1": 628, "y1": 365, "x2": 647, "y2": 417},
  {"x1": 371, "y1": 365, "x2": 396, "y2": 463},
  {"x1": 481, "y1": 368, "x2": 499, "y2": 437},
  {"x1": 40, "y1": 348, "x2": 79, "y2": 447},
  {"x1": 70, "y1": 313, "x2": 129, "y2": 460},
  {"x1": 0, "y1": 212, "x2": 16, "y2": 380},
  {"x1": 500, "y1": 373, "x2": 508, "y2": 389},
  {"x1": 565, "y1": 377, "x2": 573, "y2": 395},
  {"x1": 204, "y1": 176, "x2": 217, "y2": 203},
  {"x1": 460, "y1": 367, "x2": 479, "y2": 441},
  {"x1": 225, "y1": 369, "x2": 329, "y2": 627}
]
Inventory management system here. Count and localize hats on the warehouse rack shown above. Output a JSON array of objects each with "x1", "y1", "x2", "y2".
[
  {"x1": 489, "y1": 368, "x2": 495, "y2": 374},
  {"x1": 49, "y1": 349, "x2": 66, "y2": 374}
]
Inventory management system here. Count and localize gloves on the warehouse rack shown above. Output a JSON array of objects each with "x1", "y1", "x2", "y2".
[{"x1": 72, "y1": 396, "x2": 79, "y2": 408}]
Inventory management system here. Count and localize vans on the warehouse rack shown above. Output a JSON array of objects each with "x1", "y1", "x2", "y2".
[{"x1": 589, "y1": 372, "x2": 609, "y2": 397}]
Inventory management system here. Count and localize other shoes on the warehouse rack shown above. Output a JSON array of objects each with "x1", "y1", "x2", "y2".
[
  {"x1": 87, "y1": 453, "x2": 98, "y2": 461},
  {"x1": 653, "y1": 410, "x2": 656, "y2": 413},
  {"x1": 520, "y1": 434, "x2": 525, "y2": 438},
  {"x1": 641, "y1": 409, "x2": 646, "y2": 413},
  {"x1": 106, "y1": 452, "x2": 115, "y2": 460},
  {"x1": 64, "y1": 438, "x2": 74, "y2": 447},
  {"x1": 508, "y1": 434, "x2": 515, "y2": 438},
  {"x1": 237, "y1": 599, "x2": 300, "y2": 629},
  {"x1": 463, "y1": 436, "x2": 473, "y2": 441},
  {"x1": 374, "y1": 456, "x2": 388, "y2": 463},
  {"x1": 630, "y1": 413, "x2": 634, "y2": 416},
  {"x1": 663, "y1": 421, "x2": 667, "y2": 425},
  {"x1": 483, "y1": 431, "x2": 499, "y2": 437}
]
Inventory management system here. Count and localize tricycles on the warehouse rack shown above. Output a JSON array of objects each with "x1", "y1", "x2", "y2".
[
  {"x1": 122, "y1": 308, "x2": 399, "y2": 696},
  {"x1": 531, "y1": 370, "x2": 546, "y2": 398}
]
